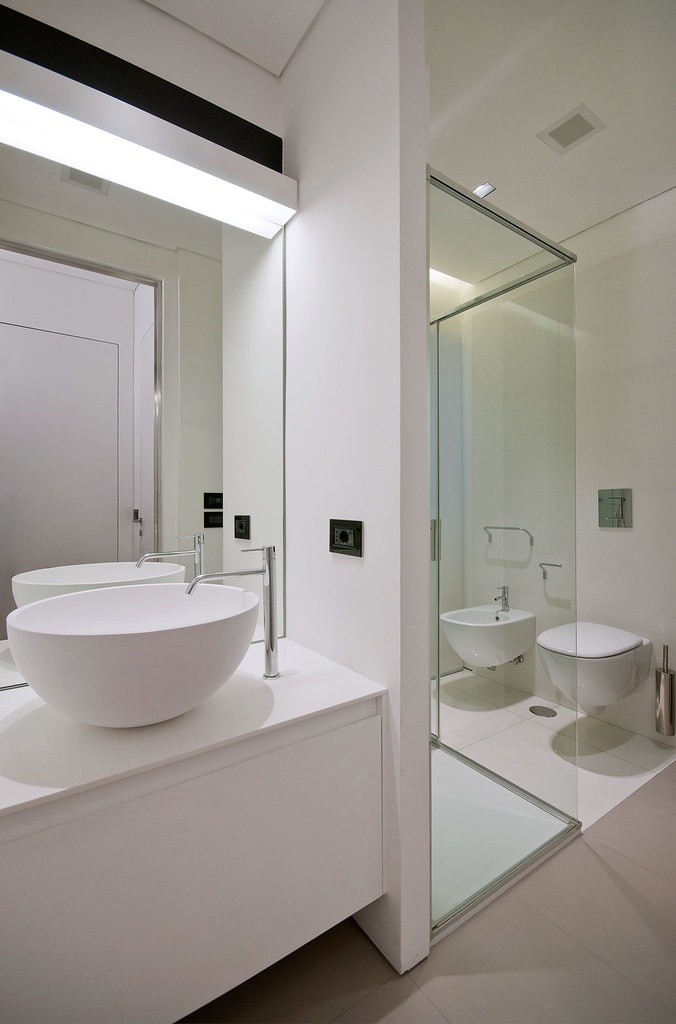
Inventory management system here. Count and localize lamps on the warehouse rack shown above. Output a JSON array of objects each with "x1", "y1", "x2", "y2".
[{"x1": 0, "y1": 50, "x2": 297, "y2": 239}]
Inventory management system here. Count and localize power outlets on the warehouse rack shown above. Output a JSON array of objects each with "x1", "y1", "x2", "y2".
[
  {"x1": 204, "y1": 512, "x2": 223, "y2": 528},
  {"x1": 204, "y1": 493, "x2": 223, "y2": 509}
]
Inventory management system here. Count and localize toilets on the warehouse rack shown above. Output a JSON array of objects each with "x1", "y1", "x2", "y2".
[{"x1": 536, "y1": 621, "x2": 652, "y2": 715}]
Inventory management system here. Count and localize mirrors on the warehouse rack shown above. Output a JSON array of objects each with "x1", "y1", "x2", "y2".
[{"x1": 0, "y1": 141, "x2": 284, "y2": 692}]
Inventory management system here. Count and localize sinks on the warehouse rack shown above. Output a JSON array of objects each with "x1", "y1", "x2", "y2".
[
  {"x1": 7, "y1": 583, "x2": 259, "y2": 727},
  {"x1": 440, "y1": 604, "x2": 536, "y2": 668},
  {"x1": 12, "y1": 562, "x2": 185, "y2": 610}
]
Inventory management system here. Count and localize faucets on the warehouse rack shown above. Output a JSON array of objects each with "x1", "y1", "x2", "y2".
[
  {"x1": 136, "y1": 535, "x2": 205, "y2": 586},
  {"x1": 185, "y1": 545, "x2": 282, "y2": 681},
  {"x1": 185, "y1": 546, "x2": 276, "y2": 595},
  {"x1": 494, "y1": 585, "x2": 510, "y2": 612}
]
